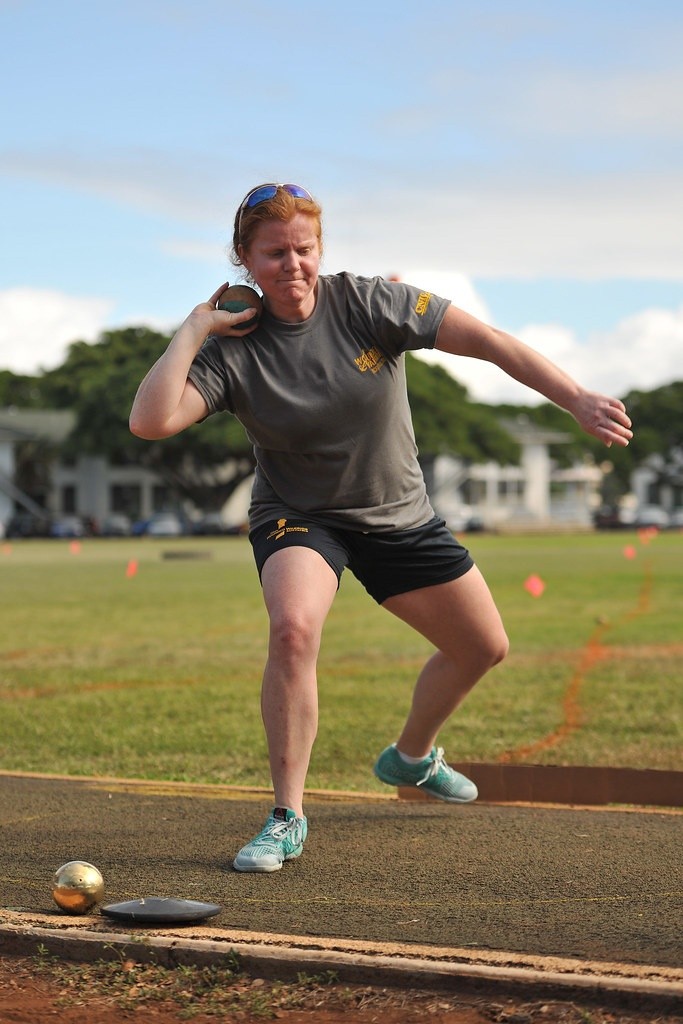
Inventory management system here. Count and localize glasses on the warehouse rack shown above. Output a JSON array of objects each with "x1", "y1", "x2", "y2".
[{"x1": 238, "y1": 183, "x2": 314, "y2": 242}]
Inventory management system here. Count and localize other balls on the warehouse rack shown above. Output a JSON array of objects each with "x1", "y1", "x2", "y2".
[
  {"x1": 51, "y1": 860, "x2": 106, "y2": 914},
  {"x1": 218, "y1": 284, "x2": 263, "y2": 329}
]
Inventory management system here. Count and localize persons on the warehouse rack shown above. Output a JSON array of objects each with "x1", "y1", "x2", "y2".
[{"x1": 129, "y1": 183, "x2": 633, "y2": 872}]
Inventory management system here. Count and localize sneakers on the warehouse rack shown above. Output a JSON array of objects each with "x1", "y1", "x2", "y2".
[
  {"x1": 374, "y1": 743, "x2": 479, "y2": 804},
  {"x1": 233, "y1": 806, "x2": 308, "y2": 871}
]
